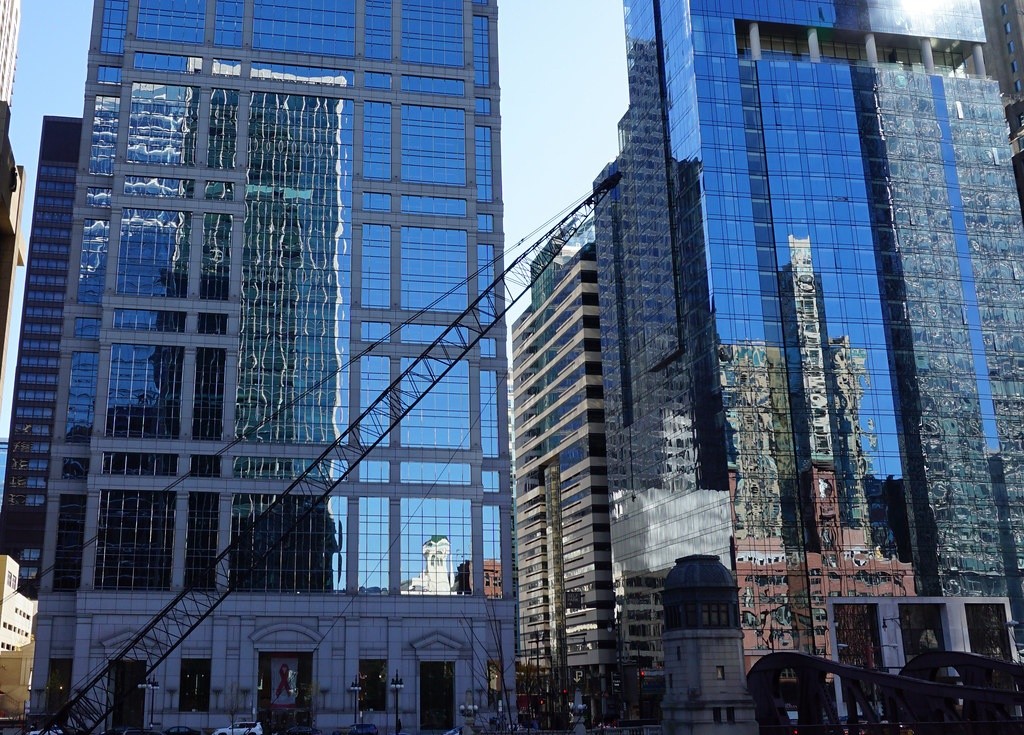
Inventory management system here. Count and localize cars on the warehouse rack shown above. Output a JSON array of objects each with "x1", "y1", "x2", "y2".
[
  {"x1": 99, "y1": 727, "x2": 162, "y2": 735},
  {"x1": 279, "y1": 725, "x2": 322, "y2": 735},
  {"x1": 162, "y1": 725, "x2": 201, "y2": 735}
]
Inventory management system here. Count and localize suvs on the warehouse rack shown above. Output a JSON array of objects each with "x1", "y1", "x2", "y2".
[
  {"x1": 333, "y1": 723, "x2": 378, "y2": 735},
  {"x1": 210, "y1": 722, "x2": 263, "y2": 735}
]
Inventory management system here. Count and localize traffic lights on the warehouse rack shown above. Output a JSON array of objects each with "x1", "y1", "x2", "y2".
[{"x1": 640, "y1": 671, "x2": 645, "y2": 678}]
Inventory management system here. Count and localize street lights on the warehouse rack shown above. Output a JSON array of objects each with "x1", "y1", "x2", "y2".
[
  {"x1": 460, "y1": 704, "x2": 478, "y2": 735},
  {"x1": 390, "y1": 669, "x2": 404, "y2": 735},
  {"x1": 882, "y1": 616, "x2": 907, "y2": 666},
  {"x1": 348, "y1": 675, "x2": 362, "y2": 724},
  {"x1": 137, "y1": 674, "x2": 159, "y2": 731}
]
